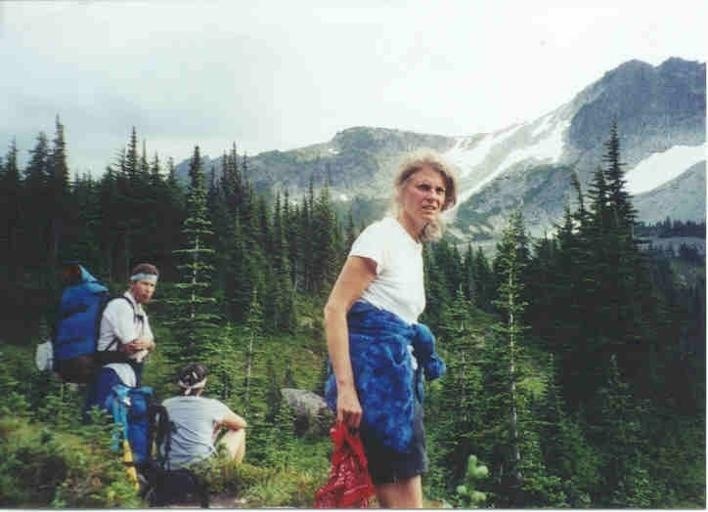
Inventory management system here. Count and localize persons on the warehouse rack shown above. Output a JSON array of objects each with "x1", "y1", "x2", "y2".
[
  {"x1": 85, "y1": 264, "x2": 161, "y2": 496},
  {"x1": 155, "y1": 362, "x2": 248, "y2": 494},
  {"x1": 322, "y1": 145, "x2": 459, "y2": 509}
]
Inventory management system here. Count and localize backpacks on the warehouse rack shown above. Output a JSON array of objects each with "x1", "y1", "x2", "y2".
[
  {"x1": 105, "y1": 384, "x2": 157, "y2": 496},
  {"x1": 53, "y1": 263, "x2": 134, "y2": 383}
]
